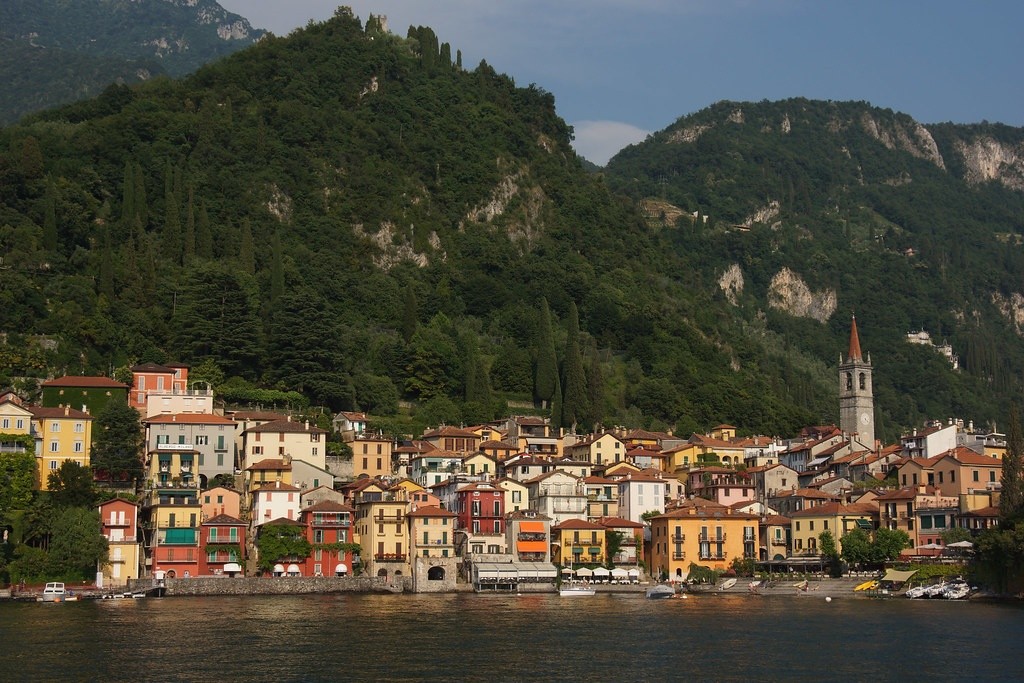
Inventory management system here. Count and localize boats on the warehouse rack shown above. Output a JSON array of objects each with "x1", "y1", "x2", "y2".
[
  {"x1": 924, "y1": 579, "x2": 949, "y2": 599},
  {"x1": 43, "y1": 582, "x2": 67, "y2": 603},
  {"x1": 559, "y1": 541, "x2": 597, "y2": 596},
  {"x1": 869, "y1": 580, "x2": 879, "y2": 590},
  {"x1": 943, "y1": 576, "x2": 971, "y2": 599},
  {"x1": 862, "y1": 580, "x2": 875, "y2": 591},
  {"x1": 905, "y1": 582, "x2": 927, "y2": 599},
  {"x1": 646, "y1": 584, "x2": 676, "y2": 600},
  {"x1": 853, "y1": 580, "x2": 872, "y2": 592}
]
